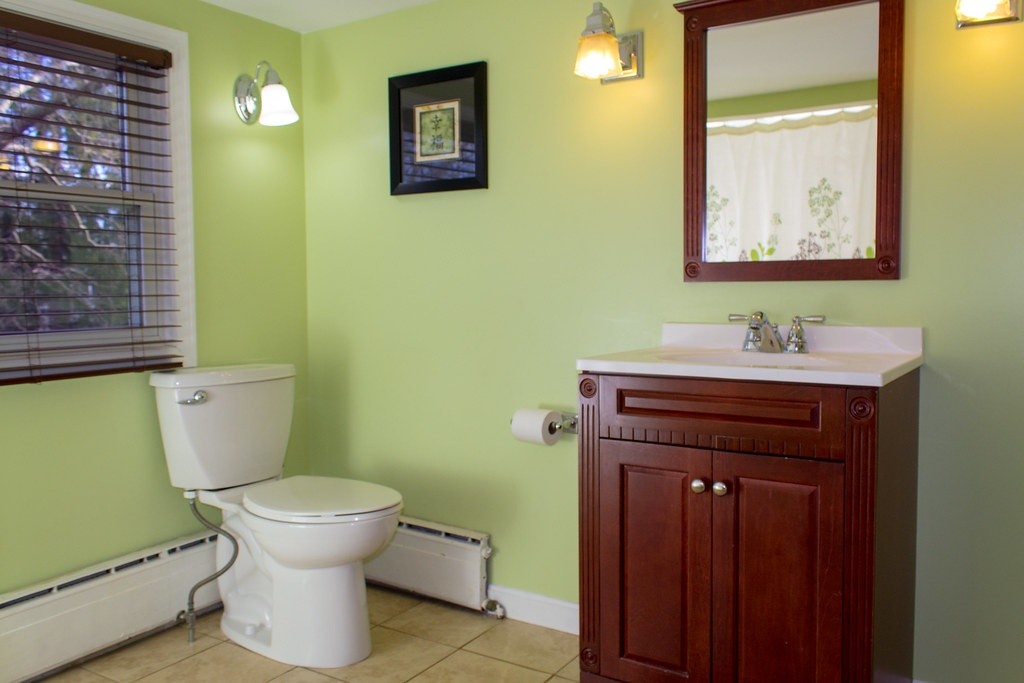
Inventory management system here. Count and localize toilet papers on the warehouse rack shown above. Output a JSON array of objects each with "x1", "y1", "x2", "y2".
[{"x1": 511, "y1": 409, "x2": 563, "y2": 445}]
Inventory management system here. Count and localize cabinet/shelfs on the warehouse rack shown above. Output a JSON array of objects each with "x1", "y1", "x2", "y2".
[{"x1": 578, "y1": 365, "x2": 920, "y2": 682}]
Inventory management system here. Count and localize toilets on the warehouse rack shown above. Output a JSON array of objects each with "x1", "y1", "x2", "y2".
[{"x1": 149, "y1": 360, "x2": 398, "y2": 667}]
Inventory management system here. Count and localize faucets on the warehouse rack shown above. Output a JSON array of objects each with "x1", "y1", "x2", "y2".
[{"x1": 749, "y1": 319, "x2": 784, "y2": 353}]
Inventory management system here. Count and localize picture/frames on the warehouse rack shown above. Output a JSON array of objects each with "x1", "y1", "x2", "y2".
[{"x1": 388, "y1": 60, "x2": 489, "y2": 196}]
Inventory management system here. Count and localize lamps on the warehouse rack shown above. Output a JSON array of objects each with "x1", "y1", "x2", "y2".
[
  {"x1": 574, "y1": 2, "x2": 646, "y2": 85},
  {"x1": 953, "y1": 0, "x2": 1021, "y2": 28},
  {"x1": 233, "y1": 59, "x2": 299, "y2": 127}
]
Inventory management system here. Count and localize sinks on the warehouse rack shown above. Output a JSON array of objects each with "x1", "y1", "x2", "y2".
[{"x1": 577, "y1": 324, "x2": 926, "y2": 387}]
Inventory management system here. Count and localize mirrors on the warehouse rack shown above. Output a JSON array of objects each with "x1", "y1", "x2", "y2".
[{"x1": 674, "y1": 0, "x2": 903, "y2": 283}]
